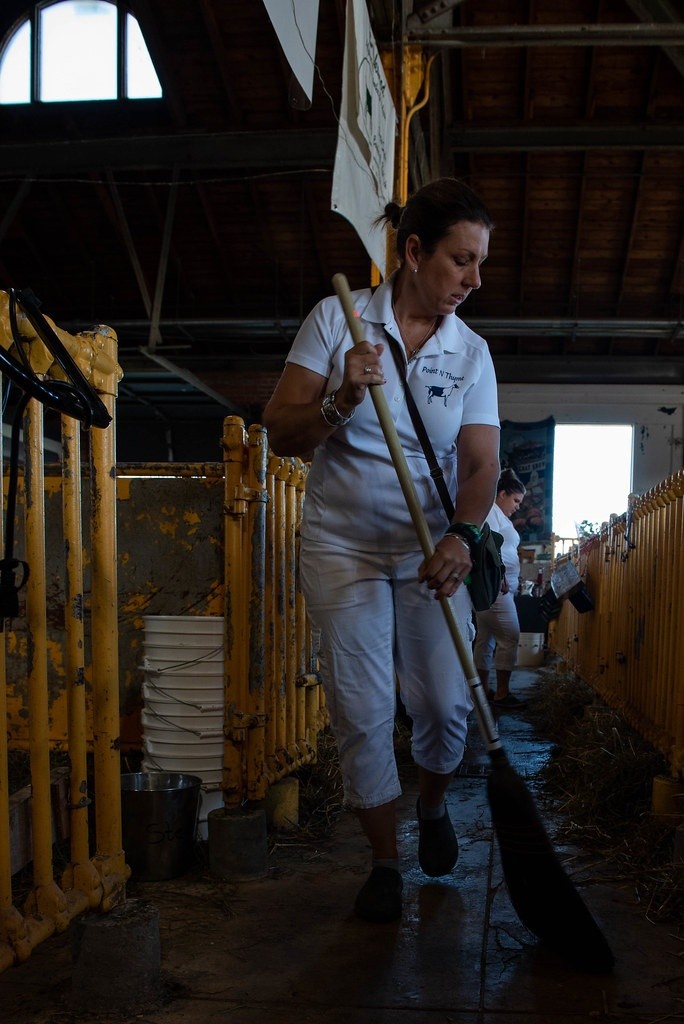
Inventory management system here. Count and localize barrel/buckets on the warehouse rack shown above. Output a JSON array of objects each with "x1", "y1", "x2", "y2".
[
  {"x1": 121, "y1": 772, "x2": 202, "y2": 881},
  {"x1": 516, "y1": 632, "x2": 547, "y2": 666},
  {"x1": 141, "y1": 616, "x2": 225, "y2": 838}
]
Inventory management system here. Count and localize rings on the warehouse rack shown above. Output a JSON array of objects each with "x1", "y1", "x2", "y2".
[
  {"x1": 364, "y1": 365, "x2": 371, "y2": 374},
  {"x1": 450, "y1": 571, "x2": 461, "y2": 582}
]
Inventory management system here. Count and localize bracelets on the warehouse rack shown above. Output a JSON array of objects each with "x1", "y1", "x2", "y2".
[
  {"x1": 320, "y1": 389, "x2": 355, "y2": 426},
  {"x1": 444, "y1": 521, "x2": 480, "y2": 551}
]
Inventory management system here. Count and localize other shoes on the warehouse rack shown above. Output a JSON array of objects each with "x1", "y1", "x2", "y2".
[
  {"x1": 486, "y1": 688, "x2": 495, "y2": 702},
  {"x1": 493, "y1": 692, "x2": 527, "y2": 707},
  {"x1": 355, "y1": 865, "x2": 403, "y2": 924},
  {"x1": 416, "y1": 796, "x2": 458, "y2": 877}
]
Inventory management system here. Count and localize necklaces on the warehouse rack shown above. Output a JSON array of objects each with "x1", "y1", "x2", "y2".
[{"x1": 393, "y1": 301, "x2": 437, "y2": 355}]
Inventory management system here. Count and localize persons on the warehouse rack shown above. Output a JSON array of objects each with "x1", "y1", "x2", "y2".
[
  {"x1": 472, "y1": 468, "x2": 527, "y2": 707},
  {"x1": 261, "y1": 177, "x2": 502, "y2": 924}
]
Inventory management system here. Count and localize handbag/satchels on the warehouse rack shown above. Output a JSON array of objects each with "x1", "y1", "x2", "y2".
[{"x1": 464, "y1": 522, "x2": 506, "y2": 611}]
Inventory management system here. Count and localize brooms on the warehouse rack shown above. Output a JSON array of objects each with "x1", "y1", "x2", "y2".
[{"x1": 330, "y1": 270, "x2": 600, "y2": 962}]
